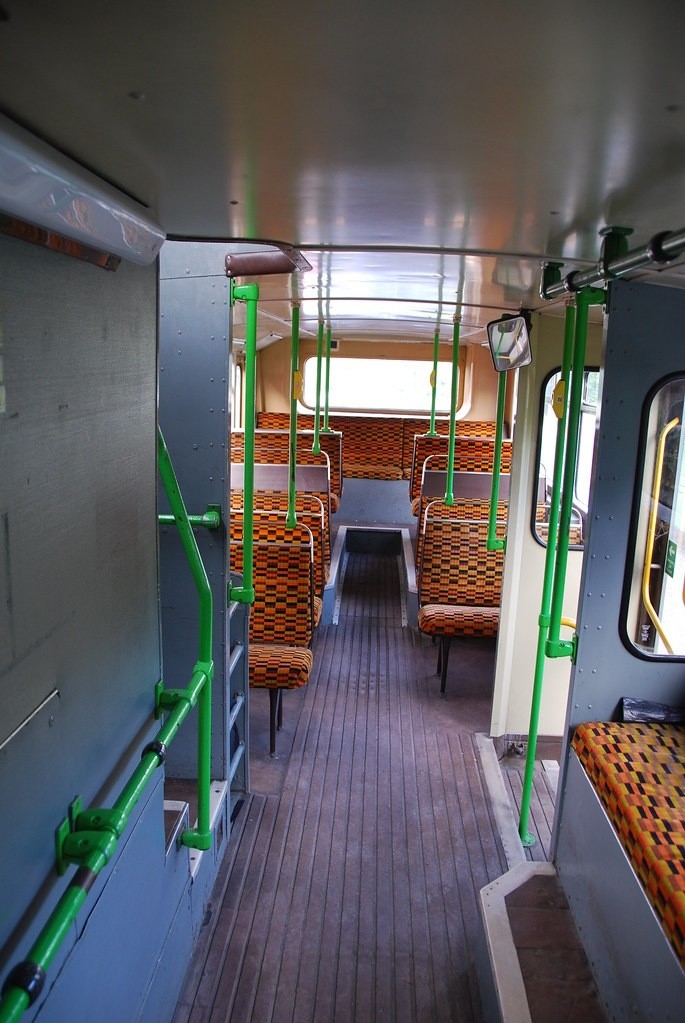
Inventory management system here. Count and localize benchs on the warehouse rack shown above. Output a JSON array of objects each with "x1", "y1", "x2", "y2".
[{"x1": 229, "y1": 412, "x2": 685, "y2": 1023}]
